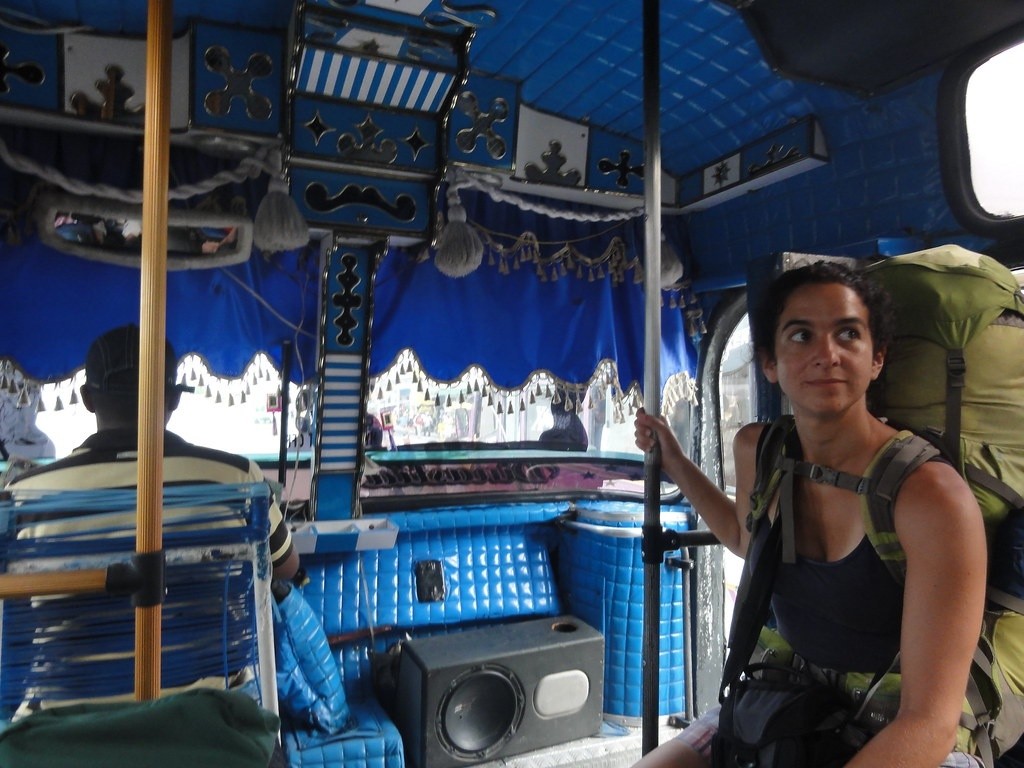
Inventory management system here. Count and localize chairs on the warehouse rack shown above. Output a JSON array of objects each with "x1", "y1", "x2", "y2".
[{"x1": 1, "y1": 483, "x2": 281, "y2": 742}]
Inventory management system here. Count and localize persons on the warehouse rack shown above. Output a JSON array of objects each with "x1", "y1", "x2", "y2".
[
  {"x1": 634, "y1": 263, "x2": 988, "y2": 768},
  {"x1": 5, "y1": 324, "x2": 301, "y2": 724}
]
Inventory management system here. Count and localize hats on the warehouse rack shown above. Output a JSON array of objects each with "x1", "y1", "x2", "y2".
[{"x1": 85, "y1": 325, "x2": 177, "y2": 397}]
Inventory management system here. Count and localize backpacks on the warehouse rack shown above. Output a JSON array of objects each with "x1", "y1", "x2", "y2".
[{"x1": 742, "y1": 249, "x2": 1024, "y2": 763}]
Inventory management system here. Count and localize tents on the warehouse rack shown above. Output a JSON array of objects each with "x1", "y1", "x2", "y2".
[{"x1": 2, "y1": 0, "x2": 1024, "y2": 757}]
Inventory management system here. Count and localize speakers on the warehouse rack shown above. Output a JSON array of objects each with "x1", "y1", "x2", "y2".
[{"x1": 392, "y1": 614, "x2": 605, "y2": 768}]
